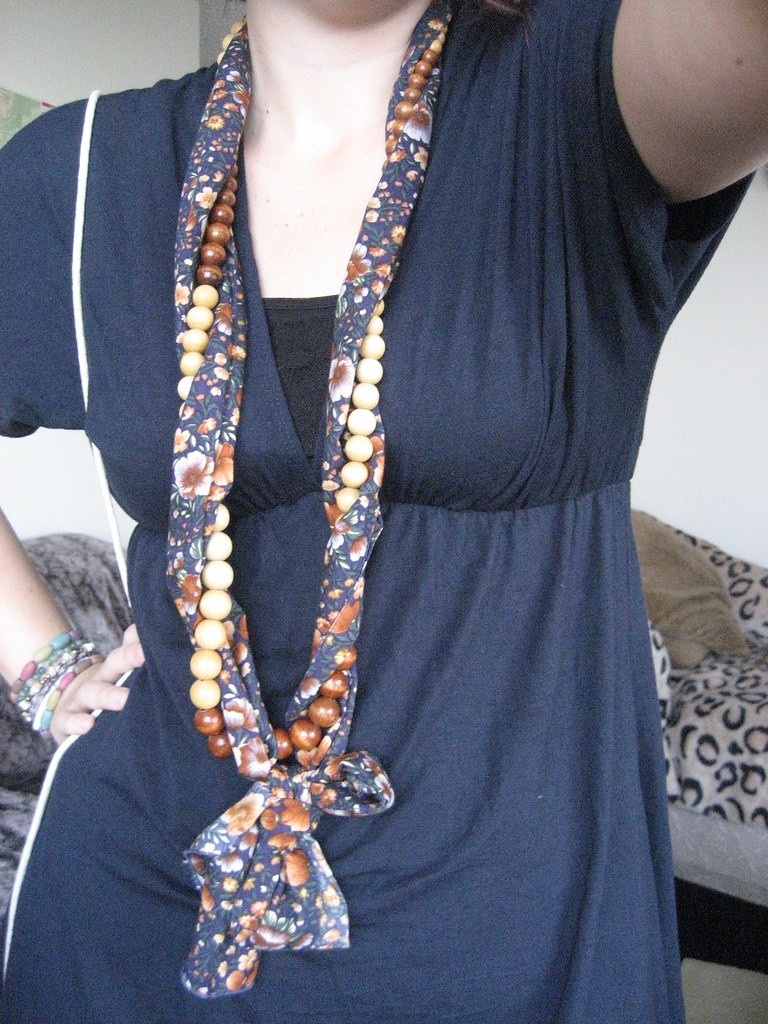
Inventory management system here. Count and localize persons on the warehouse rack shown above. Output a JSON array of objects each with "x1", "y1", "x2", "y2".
[{"x1": 0, "y1": 0, "x2": 768, "y2": 1022}]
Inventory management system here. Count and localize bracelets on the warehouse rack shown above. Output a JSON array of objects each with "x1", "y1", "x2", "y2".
[{"x1": 9, "y1": 628, "x2": 103, "y2": 741}]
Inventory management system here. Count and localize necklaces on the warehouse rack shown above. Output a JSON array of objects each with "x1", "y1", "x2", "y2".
[{"x1": 176, "y1": 24, "x2": 448, "y2": 759}]
